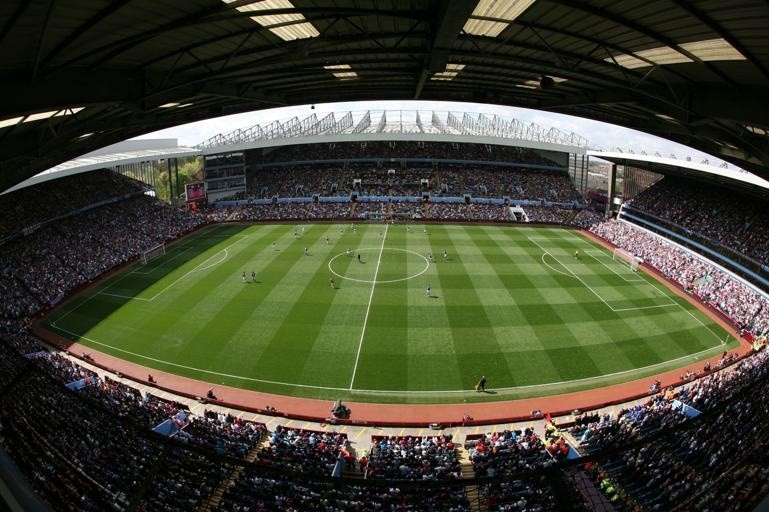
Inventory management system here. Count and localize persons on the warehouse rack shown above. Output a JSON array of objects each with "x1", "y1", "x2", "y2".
[
  {"x1": 443, "y1": 249, "x2": 448, "y2": 260},
  {"x1": 329, "y1": 277, "x2": 335, "y2": 289},
  {"x1": 574, "y1": 247, "x2": 579, "y2": 261},
  {"x1": 378, "y1": 230, "x2": 383, "y2": 240},
  {"x1": 200, "y1": 384, "x2": 769, "y2": 511},
  {"x1": 406, "y1": 225, "x2": 411, "y2": 232},
  {"x1": 590, "y1": 225, "x2": 769, "y2": 384},
  {"x1": 271, "y1": 240, "x2": 276, "y2": 252},
  {"x1": 428, "y1": 252, "x2": 432, "y2": 263},
  {"x1": 241, "y1": 271, "x2": 246, "y2": 282},
  {"x1": 250, "y1": 271, "x2": 256, "y2": 281},
  {"x1": 426, "y1": 284, "x2": 432, "y2": 296},
  {"x1": 422, "y1": 224, "x2": 427, "y2": 235},
  {"x1": 1, "y1": 224, "x2": 200, "y2": 511},
  {"x1": 476, "y1": 375, "x2": 488, "y2": 393},
  {"x1": 1, "y1": 138, "x2": 769, "y2": 225},
  {"x1": 291, "y1": 224, "x2": 362, "y2": 262}
]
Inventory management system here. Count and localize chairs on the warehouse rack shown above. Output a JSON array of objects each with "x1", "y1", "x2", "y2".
[{"x1": 0, "y1": 339, "x2": 769, "y2": 511}]
